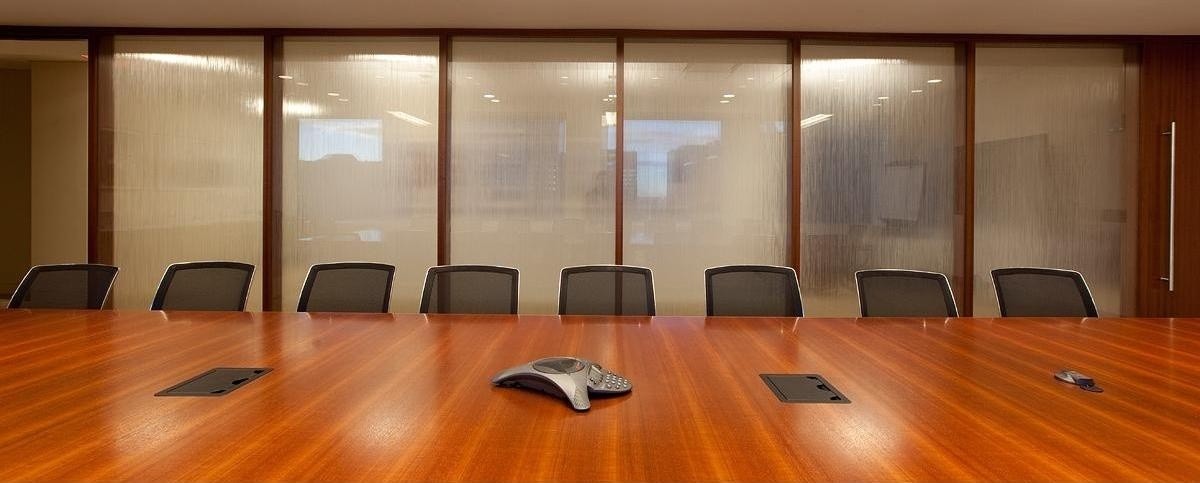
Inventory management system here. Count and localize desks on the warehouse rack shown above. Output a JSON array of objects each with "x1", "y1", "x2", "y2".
[{"x1": 0, "y1": 310, "x2": 1200, "y2": 483}]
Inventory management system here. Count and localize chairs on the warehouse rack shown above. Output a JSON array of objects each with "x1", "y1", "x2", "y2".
[
  {"x1": 558, "y1": 264, "x2": 656, "y2": 316},
  {"x1": 704, "y1": 265, "x2": 804, "y2": 317},
  {"x1": 6, "y1": 263, "x2": 119, "y2": 312},
  {"x1": 990, "y1": 268, "x2": 1100, "y2": 317},
  {"x1": 296, "y1": 263, "x2": 395, "y2": 313},
  {"x1": 419, "y1": 265, "x2": 519, "y2": 313},
  {"x1": 855, "y1": 268, "x2": 961, "y2": 318},
  {"x1": 148, "y1": 262, "x2": 255, "y2": 311}
]
